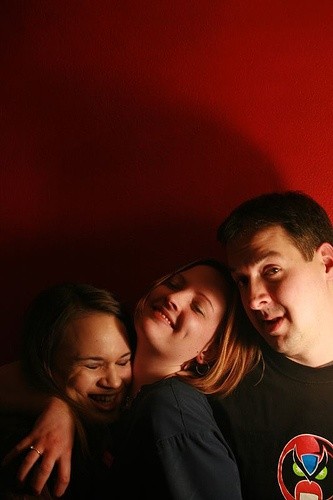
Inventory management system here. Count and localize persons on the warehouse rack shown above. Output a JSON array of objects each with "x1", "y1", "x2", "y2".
[
  {"x1": 205, "y1": 191, "x2": 333, "y2": 500},
  {"x1": 0, "y1": 280, "x2": 138, "y2": 500},
  {"x1": 0, "y1": 259, "x2": 264, "y2": 500}
]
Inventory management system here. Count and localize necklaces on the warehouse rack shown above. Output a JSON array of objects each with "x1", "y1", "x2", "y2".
[{"x1": 125, "y1": 396, "x2": 130, "y2": 408}]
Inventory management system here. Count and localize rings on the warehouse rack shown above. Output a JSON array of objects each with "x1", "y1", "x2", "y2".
[{"x1": 29, "y1": 446, "x2": 42, "y2": 459}]
